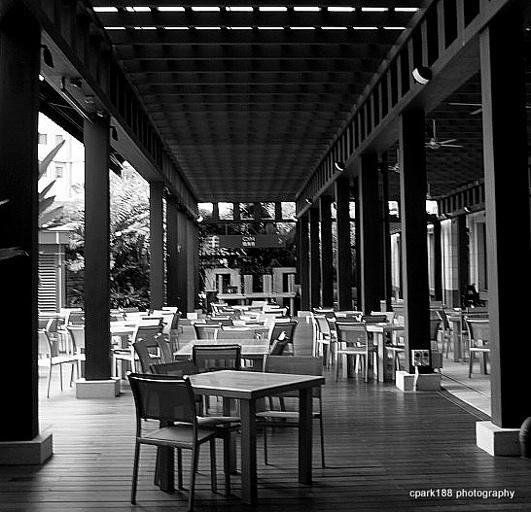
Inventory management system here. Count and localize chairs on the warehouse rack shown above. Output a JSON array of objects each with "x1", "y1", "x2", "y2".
[{"x1": 37, "y1": 289, "x2": 500, "y2": 512}]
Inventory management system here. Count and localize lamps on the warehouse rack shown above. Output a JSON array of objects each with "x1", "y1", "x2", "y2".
[
  {"x1": 69, "y1": 75, "x2": 81, "y2": 89},
  {"x1": 39, "y1": 38, "x2": 54, "y2": 68},
  {"x1": 409, "y1": 65, "x2": 431, "y2": 85},
  {"x1": 304, "y1": 198, "x2": 315, "y2": 206},
  {"x1": 109, "y1": 122, "x2": 119, "y2": 142},
  {"x1": 461, "y1": 205, "x2": 472, "y2": 215},
  {"x1": 331, "y1": 160, "x2": 346, "y2": 173},
  {"x1": 83, "y1": 92, "x2": 96, "y2": 104}
]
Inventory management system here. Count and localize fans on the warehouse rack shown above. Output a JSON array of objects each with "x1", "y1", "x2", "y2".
[
  {"x1": 422, "y1": 118, "x2": 466, "y2": 151},
  {"x1": 445, "y1": 98, "x2": 486, "y2": 115},
  {"x1": 387, "y1": 150, "x2": 401, "y2": 173}
]
